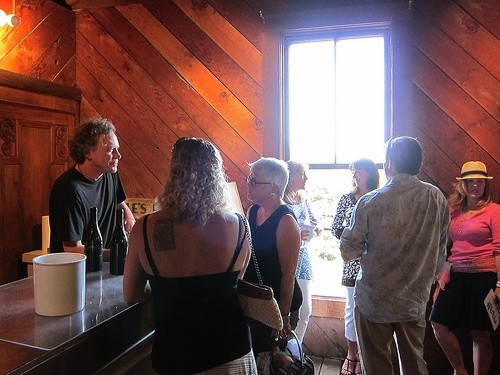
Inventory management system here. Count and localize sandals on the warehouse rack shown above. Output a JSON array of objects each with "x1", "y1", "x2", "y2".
[{"x1": 340, "y1": 358, "x2": 362, "y2": 375}]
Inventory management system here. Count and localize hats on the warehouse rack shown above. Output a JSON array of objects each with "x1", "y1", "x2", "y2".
[{"x1": 456, "y1": 161, "x2": 493, "y2": 181}]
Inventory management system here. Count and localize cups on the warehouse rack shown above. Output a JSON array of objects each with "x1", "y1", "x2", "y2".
[
  {"x1": 32, "y1": 253, "x2": 87, "y2": 316},
  {"x1": 302, "y1": 225, "x2": 315, "y2": 242}
]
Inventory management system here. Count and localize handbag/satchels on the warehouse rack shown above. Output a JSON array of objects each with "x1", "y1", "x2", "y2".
[
  {"x1": 235, "y1": 213, "x2": 284, "y2": 331},
  {"x1": 257, "y1": 329, "x2": 314, "y2": 375}
]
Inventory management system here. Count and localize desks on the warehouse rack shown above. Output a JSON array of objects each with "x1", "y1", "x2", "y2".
[{"x1": 0, "y1": 261, "x2": 155, "y2": 375}]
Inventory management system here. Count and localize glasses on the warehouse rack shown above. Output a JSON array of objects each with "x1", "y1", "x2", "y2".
[{"x1": 247, "y1": 175, "x2": 270, "y2": 187}]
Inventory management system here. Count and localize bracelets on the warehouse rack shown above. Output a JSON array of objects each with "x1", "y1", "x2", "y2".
[
  {"x1": 496, "y1": 281, "x2": 500, "y2": 287},
  {"x1": 282, "y1": 312, "x2": 291, "y2": 327}
]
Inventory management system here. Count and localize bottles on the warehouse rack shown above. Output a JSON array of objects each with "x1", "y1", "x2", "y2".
[
  {"x1": 82, "y1": 207, "x2": 103, "y2": 273},
  {"x1": 109, "y1": 209, "x2": 128, "y2": 275}
]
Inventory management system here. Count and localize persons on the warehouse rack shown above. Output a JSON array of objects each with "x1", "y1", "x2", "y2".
[
  {"x1": 245, "y1": 159, "x2": 302, "y2": 375},
  {"x1": 123, "y1": 136, "x2": 256, "y2": 375},
  {"x1": 47, "y1": 117, "x2": 137, "y2": 262},
  {"x1": 340, "y1": 136, "x2": 448, "y2": 375},
  {"x1": 429, "y1": 161, "x2": 500, "y2": 375},
  {"x1": 330, "y1": 159, "x2": 380, "y2": 375},
  {"x1": 286, "y1": 159, "x2": 324, "y2": 346}
]
